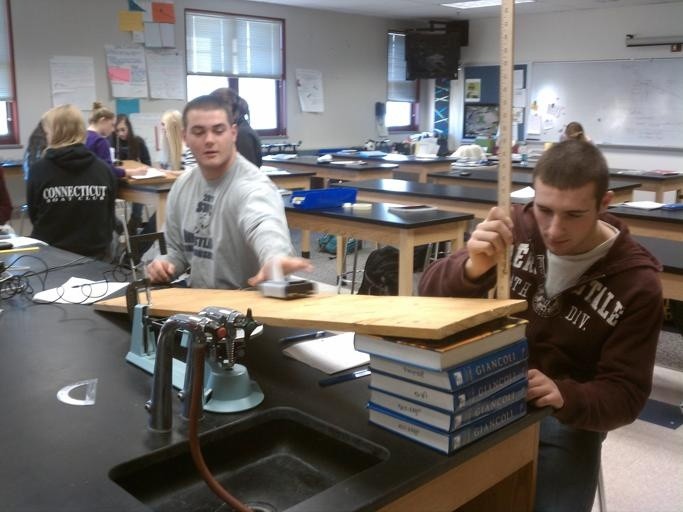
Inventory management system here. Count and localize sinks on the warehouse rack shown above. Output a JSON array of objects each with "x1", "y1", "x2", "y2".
[{"x1": 108, "y1": 407, "x2": 391, "y2": 512}]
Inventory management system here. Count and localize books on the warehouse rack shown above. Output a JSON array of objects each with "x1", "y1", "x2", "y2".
[
  {"x1": 353, "y1": 317, "x2": 530, "y2": 373},
  {"x1": 365, "y1": 399, "x2": 528, "y2": 456},
  {"x1": 366, "y1": 377, "x2": 528, "y2": 433},
  {"x1": 366, "y1": 359, "x2": 528, "y2": 414},
  {"x1": 366, "y1": 337, "x2": 529, "y2": 393},
  {"x1": 389, "y1": 201, "x2": 438, "y2": 212}
]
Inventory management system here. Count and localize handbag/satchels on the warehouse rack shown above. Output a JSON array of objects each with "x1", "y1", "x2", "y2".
[
  {"x1": 317, "y1": 235, "x2": 362, "y2": 255},
  {"x1": 358, "y1": 246, "x2": 399, "y2": 296}
]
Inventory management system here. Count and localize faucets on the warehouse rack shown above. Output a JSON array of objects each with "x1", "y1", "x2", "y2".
[{"x1": 150, "y1": 307, "x2": 262, "y2": 430}]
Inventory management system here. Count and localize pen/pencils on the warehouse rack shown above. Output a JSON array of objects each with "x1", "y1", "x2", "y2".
[
  {"x1": 72, "y1": 280, "x2": 109, "y2": 288},
  {"x1": 0, "y1": 247, "x2": 40, "y2": 253},
  {"x1": 279, "y1": 331, "x2": 325, "y2": 342},
  {"x1": 0, "y1": 244, "x2": 13, "y2": 249}
]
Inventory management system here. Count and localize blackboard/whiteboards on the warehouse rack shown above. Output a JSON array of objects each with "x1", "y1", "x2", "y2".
[{"x1": 525, "y1": 57, "x2": 683, "y2": 151}]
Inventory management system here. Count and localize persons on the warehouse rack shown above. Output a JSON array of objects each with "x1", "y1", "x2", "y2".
[
  {"x1": 416, "y1": 138, "x2": 667, "y2": 512},
  {"x1": 84, "y1": 102, "x2": 147, "y2": 176},
  {"x1": 111, "y1": 113, "x2": 151, "y2": 242},
  {"x1": 142, "y1": 110, "x2": 198, "y2": 233},
  {"x1": 210, "y1": 89, "x2": 263, "y2": 169},
  {"x1": 565, "y1": 122, "x2": 584, "y2": 139},
  {"x1": 24, "y1": 122, "x2": 47, "y2": 180},
  {"x1": 27, "y1": 104, "x2": 116, "y2": 257},
  {"x1": 147, "y1": 95, "x2": 312, "y2": 287}
]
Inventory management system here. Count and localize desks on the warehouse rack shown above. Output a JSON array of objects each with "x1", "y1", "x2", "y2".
[
  {"x1": 0, "y1": 224, "x2": 557, "y2": 509},
  {"x1": 0, "y1": 143, "x2": 681, "y2": 337}
]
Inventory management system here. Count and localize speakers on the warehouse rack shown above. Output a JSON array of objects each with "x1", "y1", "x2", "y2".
[{"x1": 446, "y1": 20, "x2": 469, "y2": 47}]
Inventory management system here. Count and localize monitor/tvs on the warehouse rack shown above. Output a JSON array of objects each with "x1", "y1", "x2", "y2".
[{"x1": 406, "y1": 35, "x2": 459, "y2": 80}]
